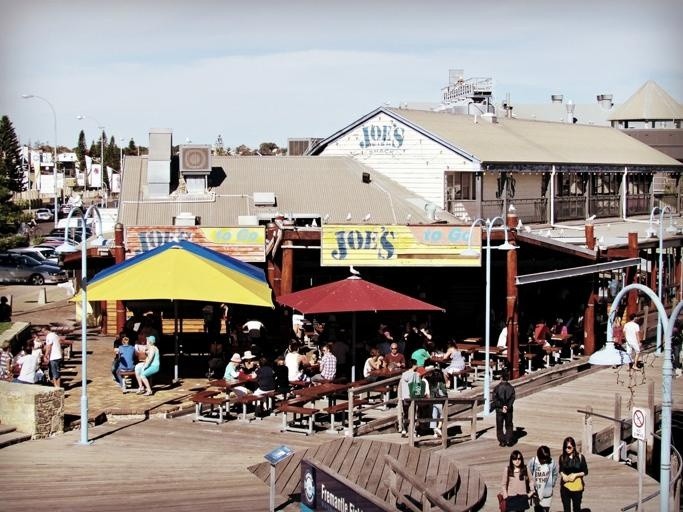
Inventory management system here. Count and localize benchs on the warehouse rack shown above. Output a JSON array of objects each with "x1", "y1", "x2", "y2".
[
  {"x1": 193, "y1": 372, "x2": 401, "y2": 434},
  {"x1": 119, "y1": 369, "x2": 148, "y2": 393},
  {"x1": 431, "y1": 333, "x2": 576, "y2": 390}
]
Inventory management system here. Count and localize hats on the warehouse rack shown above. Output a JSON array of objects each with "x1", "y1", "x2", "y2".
[
  {"x1": 274, "y1": 356, "x2": 285, "y2": 361},
  {"x1": 231, "y1": 353, "x2": 242, "y2": 363},
  {"x1": 146, "y1": 336, "x2": 155, "y2": 343},
  {"x1": 556, "y1": 319, "x2": 563, "y2": 323},
  {"x1": 241, "y1": 350, "x2": 256, "y2": 360},
  {"x1": 423, "y1": 358, "x2": 438, "y2": 367}
]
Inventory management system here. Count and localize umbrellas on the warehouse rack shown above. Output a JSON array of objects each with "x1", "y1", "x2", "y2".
[
  {"x1": 276, "y1": 275, "x2": 445, "y2": 383},
  {"x1": 67, "y1": 245, "x2": 275, "y2": 382},
  {"x1": 85, "y1": 237, "x2": 267, "y2": 333}
]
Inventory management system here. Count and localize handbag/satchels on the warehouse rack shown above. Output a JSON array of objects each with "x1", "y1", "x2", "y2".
[
  {"x1": 526, "y1": 476, "x2": 546, "y2": 501},
  {"x1": 564, "y1": 474, "x2": 583, "y2": 491},
  {"x1": 407, "y1": 382, "x2": 425, "y2": 398},
  {"x1": 498, "y1": 495, "x2": 506, "y2": 512}
]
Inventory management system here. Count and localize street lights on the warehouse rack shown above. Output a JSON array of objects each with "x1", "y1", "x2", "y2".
[
  {"x1": 586, "y1": 283, "x2": 682, "y2": 512},
  {"x1": 459, "y1": 215, "x2": 515, "y2": 416},
  {"x1": 23, "y1": 94, "x2": 59, "y2": 227},
  {"x1": 77, "y1": 114, "x2": 104, "y2": 209},
  {"x1": 646, "y1": 206, "x2": 677, "y2": 354},
  {"x1": 54, "y1": 204, "x2": 108, "y2": 444},
  {"x1": 98, "y1": 125, "x2": 122, "y2": 184}
]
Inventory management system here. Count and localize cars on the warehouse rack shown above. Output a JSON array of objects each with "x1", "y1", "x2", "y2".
[{"x1": 0, "y1": 204, "x2": 91, "y2": 284}]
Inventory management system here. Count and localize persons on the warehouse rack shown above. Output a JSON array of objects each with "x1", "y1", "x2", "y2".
[
  {"x1": 613, "y1": 317, "x2": 624, "y2": 347},
  {"x1": 527, "y1": 446, "x2": 558, "y2": 512},
  {"x1": 0, "y1": 325, "x2": 64, "y2": 388},
  {"x1": 496, "y1": 322, "x2": 525, "y2": 381},
  {"x1": 558, "y1": 437, "x2": 588, "y2": 512},
  {"x1": 492, "y1": 374, "x2": 518, "y2": 446},
  {"x1": 1, "y1": 296, "x2": 13, "y2": 323},
  {"x1": 208, "y1": 329, "x2": 351, "y2": 416},
  {"x1": 530, "y1": 308, "x2": 586, "y2": 366},
  {"x1": 109, "y1": 328, "x2": 161, "y2": 396},
  {"x1": 667, "y1": 315, "x2": 683, "y2": 369},
  {"x1": 361, "y1": 315, "x2": 438, "y2": 408},
  {"x1": 622, "y1": 313, "x2": 642, "y2": 370},
  {"x1": 501, "y1": 450, "x2": 536, "y2": 512}
]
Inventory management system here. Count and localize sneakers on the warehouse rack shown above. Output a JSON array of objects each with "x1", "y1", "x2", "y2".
[
  {"x1": 143, "y1": 390, "x2": 153, "y2": 396},
  {"x1": 398, "y1": 427, "x2": 443, "y2": 439}
]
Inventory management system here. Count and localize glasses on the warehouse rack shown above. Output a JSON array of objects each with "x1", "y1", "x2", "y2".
[
  {"x1": 136, "y1": 387, "x2": 144, "y2": 394},
  {"x1": 391, "y1": 348, "x2": 397, "y2": 350},
  {"x1": 564, "y1": 446, "x2": 571, "y2": 449},
  {"x1": 512, "y1": 457, "x2": 521, "y2": 460}
]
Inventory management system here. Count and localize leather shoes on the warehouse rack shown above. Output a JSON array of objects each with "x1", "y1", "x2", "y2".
[{"x1": 498, "y1": 440, "x2": 514, "y2": 447}]
